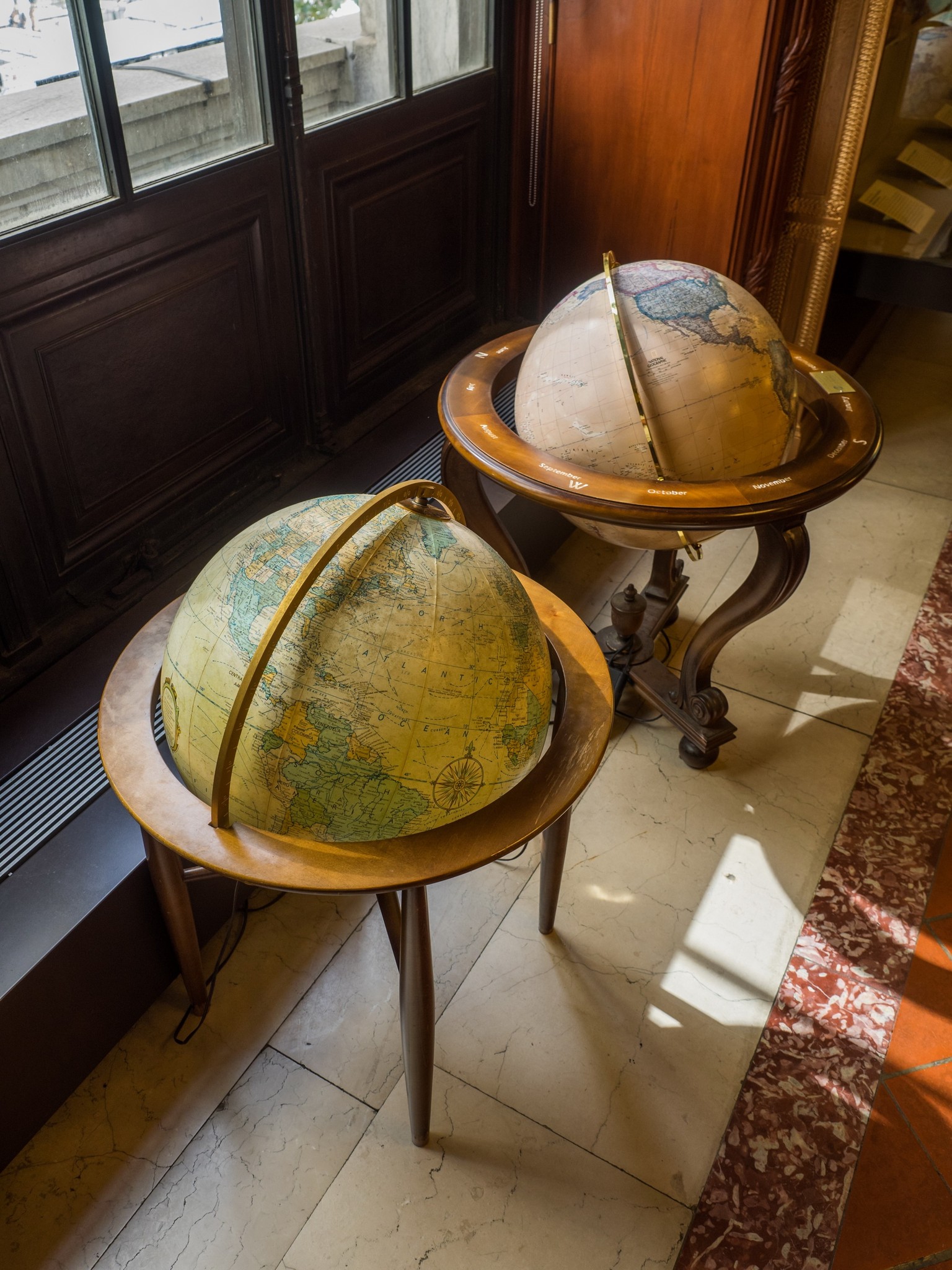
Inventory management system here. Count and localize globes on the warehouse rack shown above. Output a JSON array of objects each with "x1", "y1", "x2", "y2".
[
  {"x1": 96, "y1": 476, "x2": 614, "y2": 1145},
  {"x1": 434, "y1": 248, "x2": 884, "y2": 770}
]
